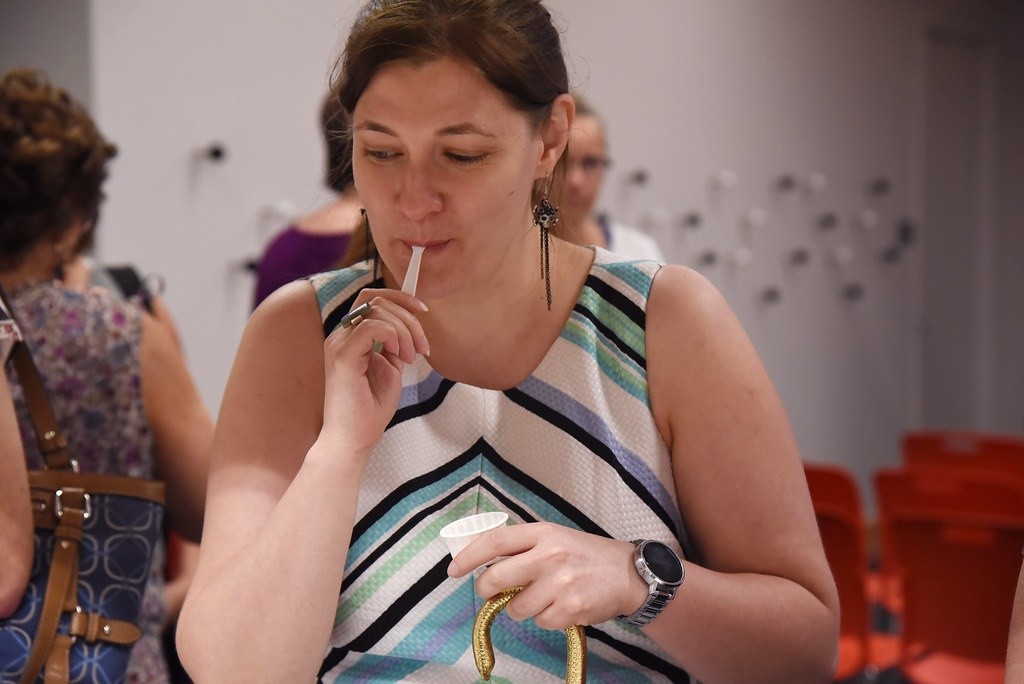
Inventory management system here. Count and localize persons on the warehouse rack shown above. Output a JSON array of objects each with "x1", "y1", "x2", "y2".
[
  {"x1": 1003, "y1": 555, "x2": 1024, "y2": 684},
  {"x1": 0, "y1": 69, "x2": 215, "y2": 684},
  {"x1": 252, "y1": 84, "x2": 662, "y2": 312},
  {"x1": 176, "y1": 0, "x2": 841, "y2": 684}
]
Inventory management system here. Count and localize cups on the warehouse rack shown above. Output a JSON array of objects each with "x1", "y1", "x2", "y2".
[{"x1": 440, "y1": 511, "x2": 508, "y2": 568}]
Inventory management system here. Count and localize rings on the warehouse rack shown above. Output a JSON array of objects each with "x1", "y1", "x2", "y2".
[{"x1": 340, "y1": 302, "x2": 373, "y2": 329}]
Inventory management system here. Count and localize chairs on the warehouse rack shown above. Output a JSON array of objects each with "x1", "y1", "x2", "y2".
[{"x1": 800, "y1": 427, "x2": 1023, "y2": 684}]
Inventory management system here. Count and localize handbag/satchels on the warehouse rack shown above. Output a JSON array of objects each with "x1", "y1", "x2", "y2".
[{"x1": 1, "y1": 292, "x2": 169, "y2": 683}]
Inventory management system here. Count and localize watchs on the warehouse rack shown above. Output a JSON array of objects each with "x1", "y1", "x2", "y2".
[{"x1": 617, "y1": 539, "x2": 685, "y2": 627}]
